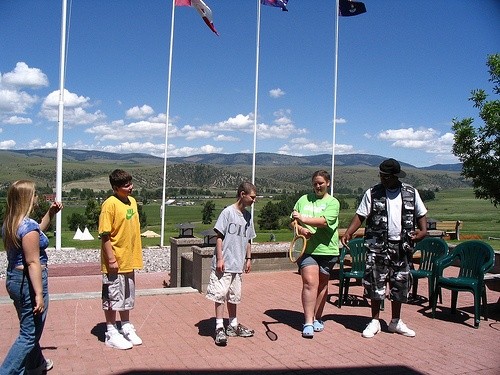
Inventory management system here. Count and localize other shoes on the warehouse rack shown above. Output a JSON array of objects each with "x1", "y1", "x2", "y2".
[
  {"x1": 313, "y1": 319, "x2": 324, "y2": 332},
  {"x1": 302, "y1": 323, "x2": 315, "y2": 337}
]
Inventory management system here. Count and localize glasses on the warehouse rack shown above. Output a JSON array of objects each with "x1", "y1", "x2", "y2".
[{"x1": 378, "y1": 174, "x2": 389, "y2": 180}]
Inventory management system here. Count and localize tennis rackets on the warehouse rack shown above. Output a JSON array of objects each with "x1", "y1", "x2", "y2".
[{"x1": 290, "y1": 209, "x2": 306, "y2": 263}]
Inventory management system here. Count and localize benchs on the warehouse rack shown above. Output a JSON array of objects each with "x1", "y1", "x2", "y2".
[{"x1": 436, "y1": 220, "x2": 464, "y2": 240}]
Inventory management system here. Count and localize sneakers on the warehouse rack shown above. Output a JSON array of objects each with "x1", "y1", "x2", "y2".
[
  {"x1": 388, "y1": 319, "x2": 416, "y2": 337},
  {"x1": 213, "y1": 326, "x2": 227, "y2": 345},
  {"x1": 226, "y1": 323, "x2": 255, "y2": 337},
  {"x1": 119, "y1": 322, "x2": 143, "y2": 345},
  {"x1": 25, "y1": 359, "x2": 53, "y2": 375},
  {"x1": 361, "y1": 319, "x2": 382, "y2": 338},
  {"x1": 104, "y1": 329, "x2": 133, "y2": 350}
]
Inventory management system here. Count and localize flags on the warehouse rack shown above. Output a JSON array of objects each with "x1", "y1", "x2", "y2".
[
  {"x1": 174, "y1": 0, "x2": 220, "y2": 36},
  {"x1": 339, "y1": 0, "x2": 367, "y2": 17},
  {"x1": 260, "y1": 0, "x2": 289, "y2": 12}
]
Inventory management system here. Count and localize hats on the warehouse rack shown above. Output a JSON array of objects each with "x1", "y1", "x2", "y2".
[{"x1": 379, "y1": 158, "x2": 406, "y2": 178}]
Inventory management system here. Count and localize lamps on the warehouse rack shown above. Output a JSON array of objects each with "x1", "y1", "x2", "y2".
[
  {"x1": 178, "y1": 222, "x2": 196, "y2": 237},
  {"x1": 426, "y1": 218, "x2": 437, "y2": 229},
  {"x1": 200, "y1": 229, "x2": 218, "y2": 246}
]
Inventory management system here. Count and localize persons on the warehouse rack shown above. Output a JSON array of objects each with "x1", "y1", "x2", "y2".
[
  {"x1": 289, "y1": 170, "x2": 340, "y2": 336},
  {"x1": 342, "y1": 159, "x2": 427, "y2": 338},
  {"x1": 213, "y1": 182, "x2": 257, "y2": 346},
  {"x1": 0, "y1": 180, "x2": 63, "y2": 375},
  {"x1": 101, "y1": 168, "x2": 143, "y2": 350}
]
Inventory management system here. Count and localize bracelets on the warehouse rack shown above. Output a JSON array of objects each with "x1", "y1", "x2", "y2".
[
  {"x1": 245, "y1": 257, "x2": 252, "y2": 260},
  {"x1": 299, "y1": 228, "x2": 302, "y2": 233}
]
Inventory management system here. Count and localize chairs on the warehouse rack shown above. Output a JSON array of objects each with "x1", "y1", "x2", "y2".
[
  {"x1": 339, "y1": 239, "x2": 384, "y2": 311},
  {"x1": 432, "y1": 241, "x2": 495, "y2": 328},
  {"x1": 407, "y1": 237, "x2": 448, "y2": 306}
]
